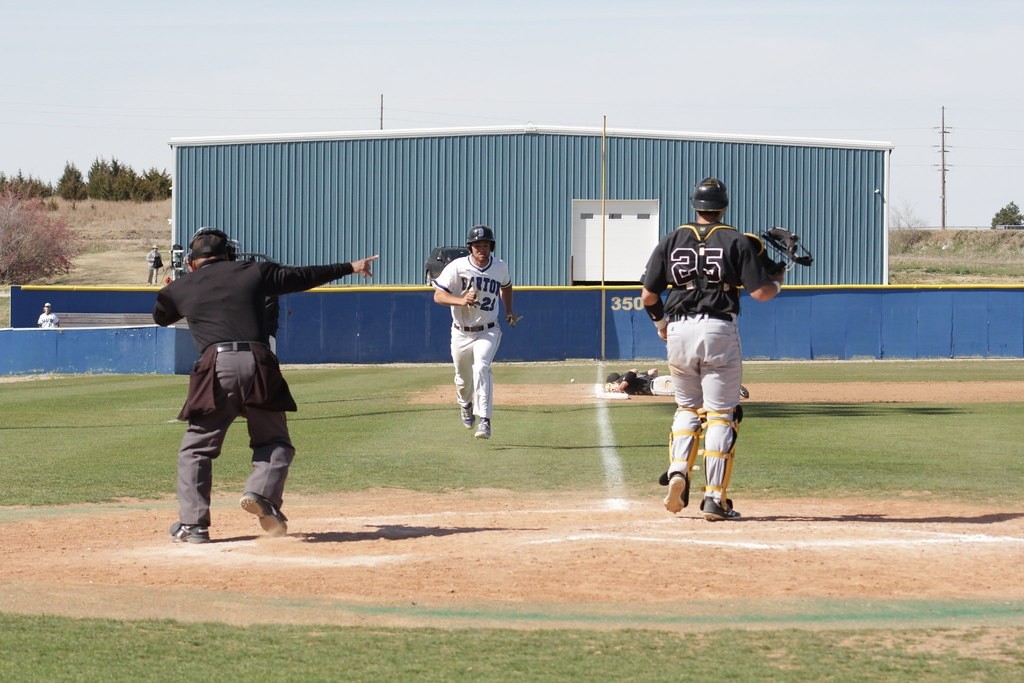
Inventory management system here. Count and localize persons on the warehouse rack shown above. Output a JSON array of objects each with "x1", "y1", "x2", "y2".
[
  {"x1": 146, "y1": 243, "x2": 161, "y2": 283},
  {"x1": 37, "y1": 302, "x2": 60, "y2": 327},
  {"x1": 640, "y1": 177, "x2": 783, "y2": 522},
  {"x1": 154, "y1": 226, "x2": 380, "y2": 538},
  {"x1": 431, "y1": 224, "x2": 518, "y2": 437},
  {"x1": 607, "y1": 370, "x2": 749, "y2": 398}
]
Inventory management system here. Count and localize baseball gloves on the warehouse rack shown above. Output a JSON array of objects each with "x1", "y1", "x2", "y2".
[{"x1": 605, "y1": 382, "x2": 621, "y2": 393}]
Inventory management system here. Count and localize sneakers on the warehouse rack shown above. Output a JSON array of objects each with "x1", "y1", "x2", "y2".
[
  {"x1": 703, "y1": 496, "x2": 741, "y2": 521},
  {"x1": 475, "y1": 417, "x2": 491, "y2": 439},
  {"x1": 240, "y1": 492, "x2": 288, "y2": 536},
  {"x1": 663, "y1": 473, "x2": 686, "y2": 514},
  {"x1": 461, "y1": 404, "x2": 475, "y2": 429},
  {"x1": 169, "y1": 521, "x2": 210, "y2": 543}
]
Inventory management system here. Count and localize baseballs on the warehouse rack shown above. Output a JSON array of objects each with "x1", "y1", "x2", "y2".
[{"x1": 570, "y1": 378, "x2": 575, "y2": 383}]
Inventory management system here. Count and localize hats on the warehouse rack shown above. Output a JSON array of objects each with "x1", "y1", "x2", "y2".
[
  {"x1": 153, "y1": 245, "x2": 158, "y2": 249},
  {"x1": 45, "y1": 303, "x2": 51, "y2": 307},
  {"x1": 606, "y1": 373, "x2": 620, "y2": 383}
]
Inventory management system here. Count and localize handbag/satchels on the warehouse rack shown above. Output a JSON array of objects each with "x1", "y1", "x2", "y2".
[
  {"x1": 243, "y1": 341, "x2": 297, "y2": 412},
  {"x1": 177, "y1": 345, "x2": 218, "y2": 421}
]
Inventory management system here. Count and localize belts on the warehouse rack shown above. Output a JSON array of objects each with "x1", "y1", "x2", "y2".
[
  {"x1": 454, "y1": 322, "x2": 494, "y2": 332},
  {"x1": 215, "y1": 343, "x2": 251, "y2": 353},
  {"x1": 668, "y1": 312, "x2": 733, "y2": 322},
  {"x1": 651, "y1": 381, "x2": 653, "y2": 389}
]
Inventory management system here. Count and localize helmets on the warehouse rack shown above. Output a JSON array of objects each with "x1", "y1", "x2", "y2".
[
  {"x1": 690, "y1": 177, "x2": 728, "y2": 211},
  {"x1": 466, "y1": 225, "x2": 495, "y2": 253}
]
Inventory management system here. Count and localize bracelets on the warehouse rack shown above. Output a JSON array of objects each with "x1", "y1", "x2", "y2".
[
  {"x1": 774, "y1": 280, "x2": 782, "y2": 293},
  {"x1": 654, "y1": 317, "x2": 668, "y2": 330}
]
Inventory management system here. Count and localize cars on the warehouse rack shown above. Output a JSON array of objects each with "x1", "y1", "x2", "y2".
[{"x1": 424, "y1": 245, "x2": 472, "y2": 286}]
